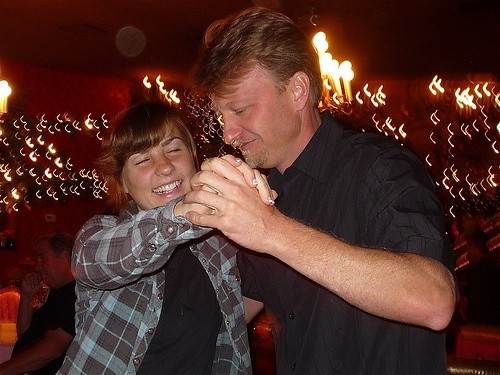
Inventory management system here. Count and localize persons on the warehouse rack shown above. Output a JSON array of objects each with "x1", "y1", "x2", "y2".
[
  {"x1": 181, "y1": 6, "x2": 457, "y2": 375},
  {"x1": 52, "y1": 97, "x2": 275, "y2": 375},
  {"x1": 1, "y1": 233, "x2": 76, "y2": 374}
]
